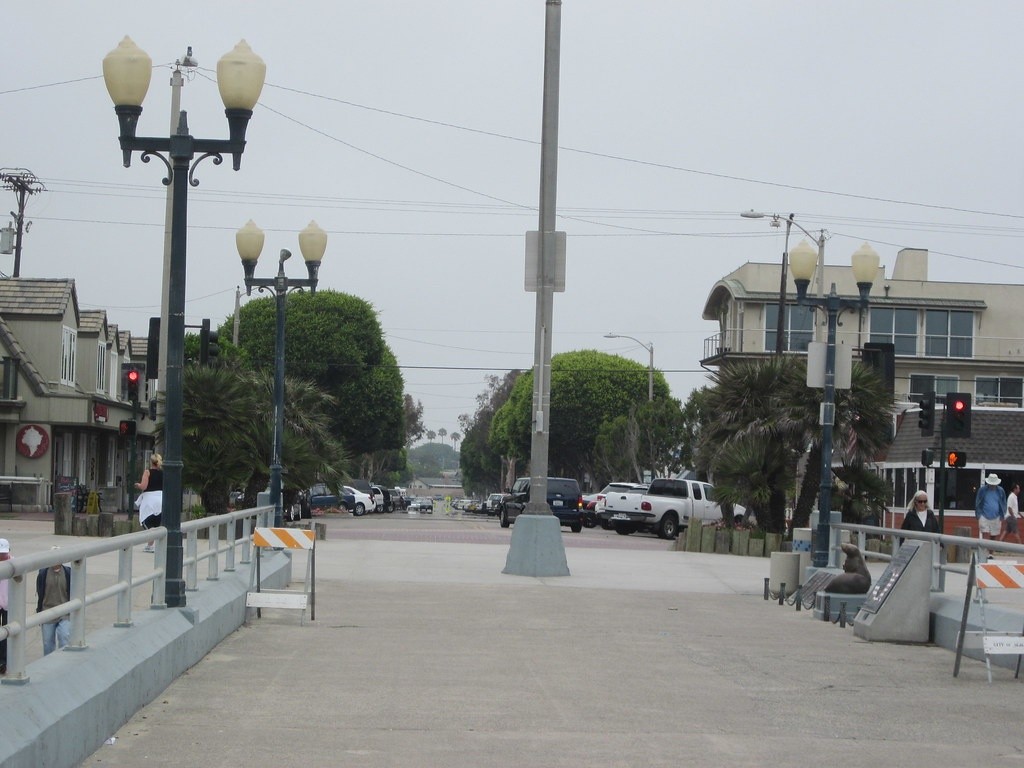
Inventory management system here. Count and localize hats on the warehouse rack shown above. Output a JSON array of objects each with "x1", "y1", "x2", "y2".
[
  {"x1": 0, "y1": 538, "x2": 11, "y2": 554},
  {"x1": 985, "y1": 473, "x2": 1002, "y2": 486}
]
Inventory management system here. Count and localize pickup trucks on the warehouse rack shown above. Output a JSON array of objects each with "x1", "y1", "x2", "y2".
[{"x1": 596, "y1": 479, "x2": 747, "y2": 539}]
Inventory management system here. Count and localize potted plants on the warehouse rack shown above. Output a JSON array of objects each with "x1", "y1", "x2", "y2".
[{"x1": 715, "y1": 302, "x2": 732, "y2": 355}]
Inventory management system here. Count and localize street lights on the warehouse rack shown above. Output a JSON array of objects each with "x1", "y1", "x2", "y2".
[
  {"x1": 740, "y1": 211, "x2": 825, "y2": 342},
  {"x1": 604, "y1": 334, "x2": 655, "y2": 482},
  {"x1": 236, "y1": 219, "x2": 328, "y2": 550},
  {"x1": 790, "y1": 238, "x2": 879, "y2": 567},
  {"x1": 102, "y1": 35, "x2": 266, "y2": 609}
]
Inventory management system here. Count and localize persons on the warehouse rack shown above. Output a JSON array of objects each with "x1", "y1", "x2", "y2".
[
  {"x1": 899, "y1": 491, "x2": 944, "y2": 551},
  {"x1": 1000, "y1": 484, "x2": 1023, "y2": 544},
  {"x1": 135, "y1": 454, "x2": 163, "y2": 551},
  {"x1": 0, "y1": 538, "x2": 16, "y2": 676},
  {"x1": 36, "y1": 546, "x2": 71, "y2": 657},
  {"x1": 975, "y1": 473, "x2": 1006, "y2": 559}
]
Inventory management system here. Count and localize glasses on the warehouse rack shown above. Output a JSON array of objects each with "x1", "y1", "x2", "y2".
[{"x1": 915, "y1": 499, "x2": 928, "y2": 504}]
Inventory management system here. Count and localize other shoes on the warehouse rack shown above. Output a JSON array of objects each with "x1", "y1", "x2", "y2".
[
  {"x1": 988, "y1": 554, "x2": 994, "y2": 559},
  {"x1": 141, "y1": 545, "x2": 155, "y2": 553}
]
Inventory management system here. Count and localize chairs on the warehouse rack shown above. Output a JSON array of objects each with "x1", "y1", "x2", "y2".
[{"x1": 77, "y1": 485, "x2": 104, "y2": 513}]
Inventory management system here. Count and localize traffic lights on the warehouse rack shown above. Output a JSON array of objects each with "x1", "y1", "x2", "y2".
[
  {"x1": 922, "y1": 449, "x2": 933, "y2": 466},
  {"x1": 128, "y1": 370, "x2": 138, "y2": 401},
  {"x1": 200, "y1": 319, "x2": 219, "y2": 363},
  {"x1": 948, "y1": 451, "x2": 966, "y2": 467},
  {"x1": 918, "y1": 392, "x2": 935, "y2": 437},
  {"x1": 119, "y1": 421, "x2": 136, "y2": 436},
  {"x1": 945, "y1": 392, "x2": 971, "y2": 438}
]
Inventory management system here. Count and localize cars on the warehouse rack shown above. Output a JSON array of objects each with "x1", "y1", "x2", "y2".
[
  {"x1": 496, "y1": 477, "x2": 585, "y2": 533},
  {"x1": 582, "y1": 482, "x2": 651, "y2": 527},
  {"x1": 236, "y1": 484, "x2": 355, "y2": 522},
  {"x1": 450, "y1": 494, "x2": 505, "y2": 515},
  {"x1": 343, "y1": 479, "x2": 443, "y2": 513}
]
